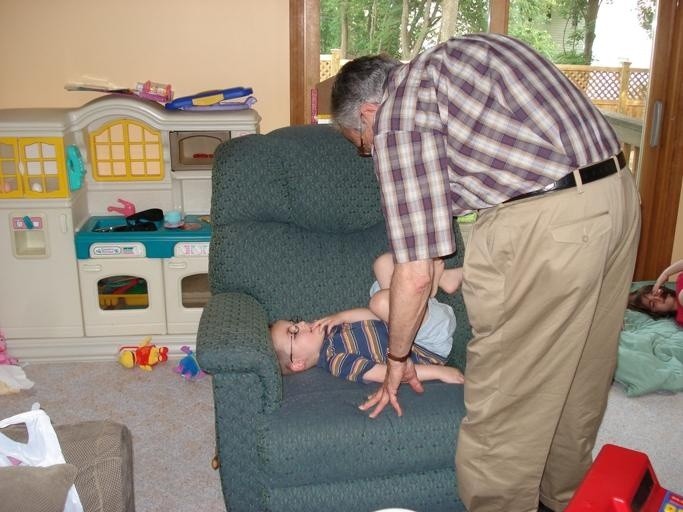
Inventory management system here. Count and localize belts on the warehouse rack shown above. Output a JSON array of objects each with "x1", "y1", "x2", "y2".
[{"x1": 479, "y1": 148, "x2": 628, "y2": 205}]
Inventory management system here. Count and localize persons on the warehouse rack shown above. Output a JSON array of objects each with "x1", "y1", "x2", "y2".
[
  {"x1": 627, "y1": 258, "x2": 683, "y2": 328},
  {"x1": 330, "y1": 30, "x2": 642, "y2": 511},
  {"x1": 270, "y1": 253, "x2": 464, "y2": 385}
]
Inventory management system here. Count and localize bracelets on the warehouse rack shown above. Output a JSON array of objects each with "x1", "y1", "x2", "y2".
[{"x1": 385, "y1": 349, "x2": 412, "y2": 363}]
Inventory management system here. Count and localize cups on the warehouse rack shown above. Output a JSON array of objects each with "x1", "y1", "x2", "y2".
[{"x1": 164, "y1": 210, "x2": 181, "y2": 224}]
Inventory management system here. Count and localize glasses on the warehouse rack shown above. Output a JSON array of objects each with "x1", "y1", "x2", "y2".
[
  {"x1": 287, "y1": 315, "x2": 302, "y2": 365},
  {"x1": 356, "y1": 114, "x2": 373, "y2": 159}
]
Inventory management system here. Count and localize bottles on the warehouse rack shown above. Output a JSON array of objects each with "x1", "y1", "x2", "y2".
[{"x1": 135, "y1": 80, "x2": 172, "y2": 98}]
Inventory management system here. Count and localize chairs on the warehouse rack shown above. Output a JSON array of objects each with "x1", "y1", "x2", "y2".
[
  {"x1": 0, "y1": 422, "x2": 136, "y2": 512},
  {"x1": 196, "y1": 124, "x2": 472, "y2": 512}
]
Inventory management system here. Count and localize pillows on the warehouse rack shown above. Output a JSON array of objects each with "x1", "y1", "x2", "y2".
[{"x1": 0, "y1": 464, "x2": 78, "y2": 511}]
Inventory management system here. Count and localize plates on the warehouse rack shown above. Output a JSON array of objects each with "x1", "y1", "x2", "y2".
[{"x1": 165, "y1": 223, "x2": 184, "y2": 228}]
